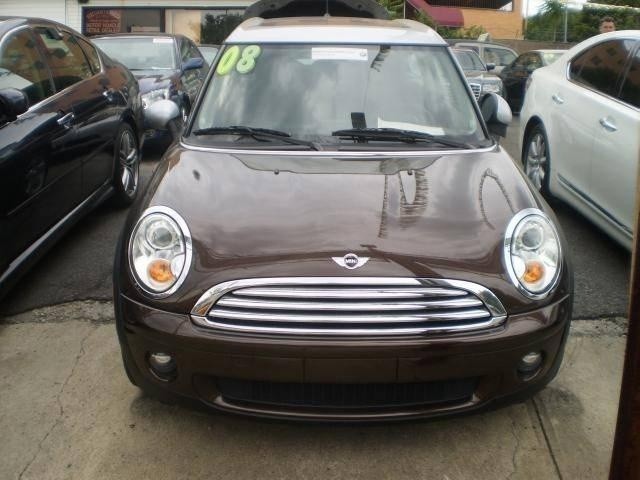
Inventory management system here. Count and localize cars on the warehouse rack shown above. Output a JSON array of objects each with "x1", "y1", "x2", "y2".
[
  {"x1": 110, "y1": 14, "x2": 577, "y2": 428},
  {"x1": 410, "y1": 48, "x2": 504, "y2": 105},
  {"x1": 89, "y1": 32, "x2": 211, "y2": 148},
  {"x1": 0, "y1": 14, "x2": 146, "y2": 301},
  {"x1": 516, "y1": 29, "x2": 640, "y2": 255},
  {"x1": 497, "y1": 50, "x2": 570, "y2": 112}
]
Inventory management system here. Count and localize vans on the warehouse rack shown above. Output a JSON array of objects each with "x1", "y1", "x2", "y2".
[{"x1": 452, "y1": 40, "x2": 520, "y2": 76}]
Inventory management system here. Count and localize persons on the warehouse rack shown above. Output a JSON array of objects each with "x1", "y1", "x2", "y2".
[{"x1": 599, "y1": 16, "x2": 617, "y2": 33}]
